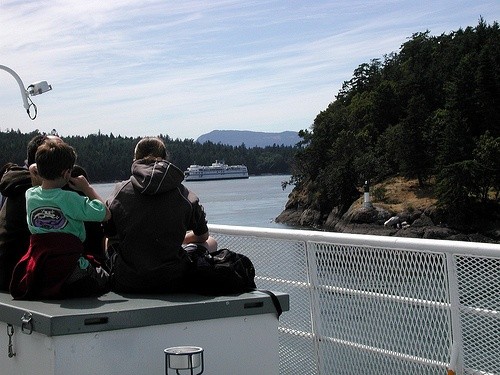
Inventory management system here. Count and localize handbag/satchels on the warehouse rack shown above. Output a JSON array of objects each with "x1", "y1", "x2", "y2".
[{"x1": 168, "y1": 243, "x2": 257, "y2": 294}]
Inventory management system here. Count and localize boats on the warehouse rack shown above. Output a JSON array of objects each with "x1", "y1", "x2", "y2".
[{"x1": 183, "y1": 160, "x2": 249, "y2": 180}]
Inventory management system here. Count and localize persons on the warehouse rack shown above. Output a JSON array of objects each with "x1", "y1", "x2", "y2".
[
  {"x1": 9, "y1": 140, "x2": 112, "y2": 301},
  {"x1": 62, "y1": 166, "x2": 111, "y2": 275},
  {"x1": 1, "y1": 134, "x2": 66, "y2": 294},
  {"x1": 1, "y1": 135, "x2": 49, "y2": 211},
  {"x1": 104, "y1": 136, "x2": 219, "y2": 295}
]
